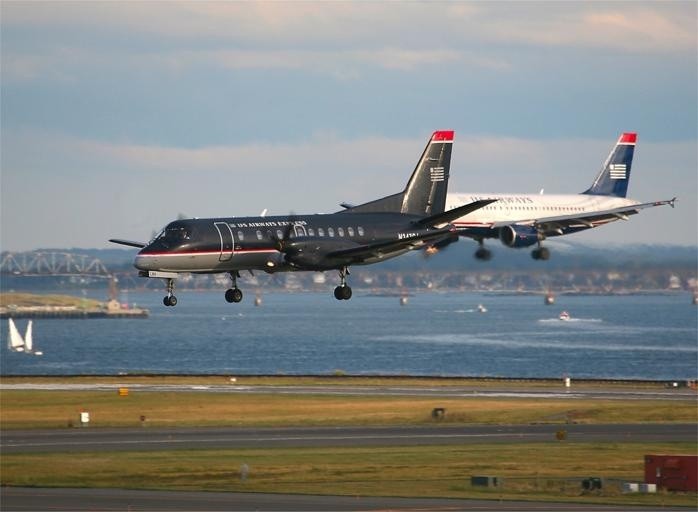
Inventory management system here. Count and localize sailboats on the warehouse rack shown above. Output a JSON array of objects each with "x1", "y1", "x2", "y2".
[{"x1": 5, "y1": 314, "x2": 43, "y2": 357}]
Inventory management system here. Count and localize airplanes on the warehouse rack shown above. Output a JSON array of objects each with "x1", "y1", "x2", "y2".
[
  {"x1": 107, "y1": 126, "x2": 501, "y2": 309},
  {"x1": 435, "y1": 126, "x2": 677, "y2": 264}
]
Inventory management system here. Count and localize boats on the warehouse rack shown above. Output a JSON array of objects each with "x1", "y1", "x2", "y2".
[
  {"x1": 474, "y1": 302, "x2": 488, "y2": 313},
  {"x1": 557, "y1": 310, "x2": 572, "y2": 322}
]
